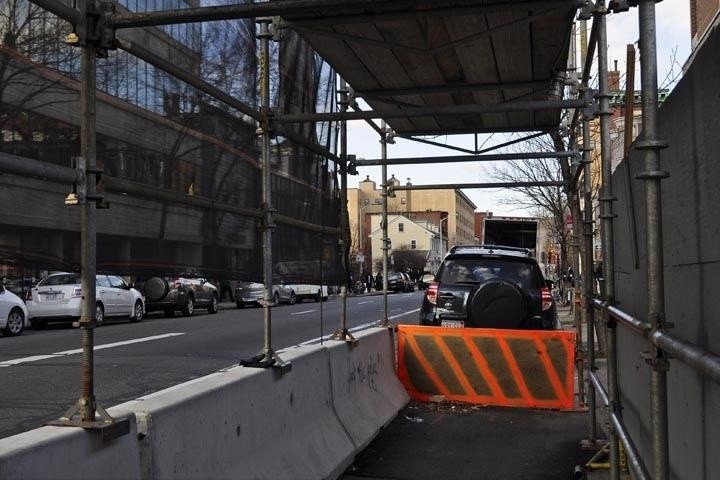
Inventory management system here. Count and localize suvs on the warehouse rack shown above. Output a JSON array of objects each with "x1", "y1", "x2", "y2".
[
  {"x1": 134, "y1": 262, "x2": 219, "y2": 318},
  {"x1": 418, "y1": 242, "x2": 557, "y2": 333}
]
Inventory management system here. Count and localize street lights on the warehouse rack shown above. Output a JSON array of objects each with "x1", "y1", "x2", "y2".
[
  {"x1": 439, "y1": 212, "x2": 460, "y2": 263},
  {"x1": 360, "y1": 202, "x2": 378, "y2": 281}
]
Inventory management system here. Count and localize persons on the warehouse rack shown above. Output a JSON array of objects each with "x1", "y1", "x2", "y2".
[
  {"x1": 596, "y1": 263, "x2": 603, "y2": 281},
  {"x1": 364, "y1": 272, "x2": 382, "y2": 293}
]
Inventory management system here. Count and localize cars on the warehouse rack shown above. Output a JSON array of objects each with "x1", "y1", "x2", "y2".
[
  {"x1": 235, "y1": 272, "x2": 434, "y2": 307},
  {"x1": 24, "y1": 271, "x2": 148, "y2": 328},
  {"x1": 0, "y1": 281, "x2": 29, "y2": 337}
]
